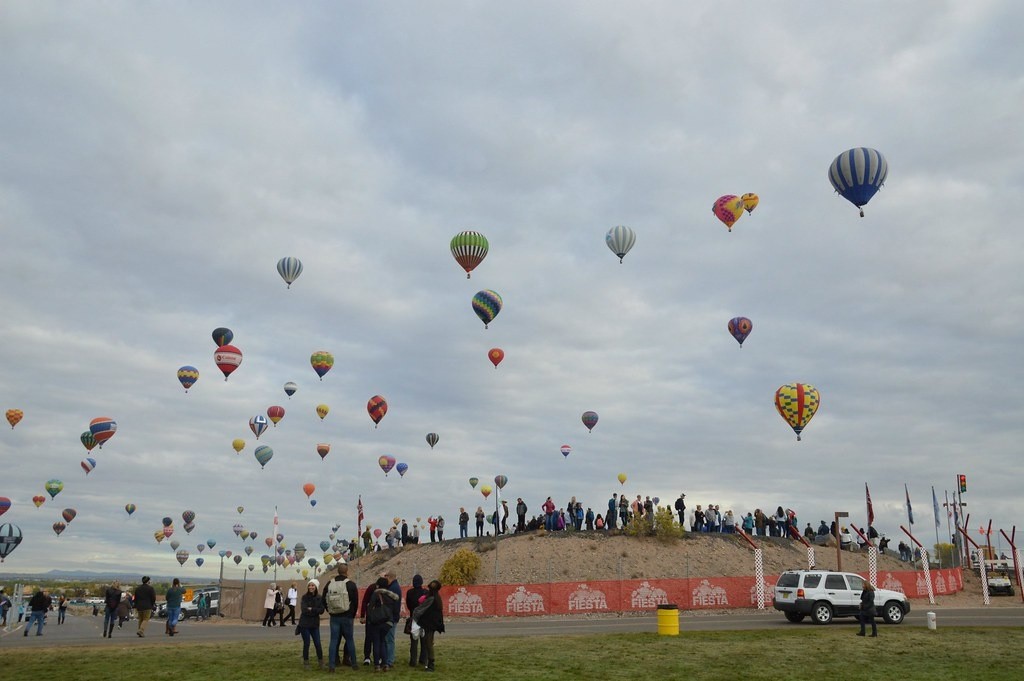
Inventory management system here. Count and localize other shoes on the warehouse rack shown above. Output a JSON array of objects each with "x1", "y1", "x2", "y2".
[
  {"x1": 335, "y1": 658, "x2": 340, "y2": 666},
  {"x1": 363, "y1": 658, "x2": 370, "y2": 666},
  {"x1": 869, "y1": 634, "x2": 877, "y2": 637},
  {"x1": 23, "y1": 630, "x2": 28, "y2": 636},
  {"x1": 101, "y1": 622, "x2": 178, "y2": 638},
  {"x1": 383, "y1": 665, "x2": 389, "y2": 672},
  {"x1": 37, "y1": 632, "x2": 42, "y2": 636},
  {"x1": 304, "y1": 660, "x2": 309, "y2": 670},
  {"x1": 375, "y1": 665, "x2": 380, "y2": 672},
  {"x1": 318, "y1": 658, "x2": 324, "y2": 669},
  {"x1": 856, "y1": 633, "x2": 865, "y2": 636},
  {"x1": 352, "y1": 664, "x2": 358, "y2": 670},
  {"x1": 262, "y1": 620, "x2": 297, "y2": 627},
  {"x1": 342, "y1": 659, "x2": 352, "y2": 667},
  {"x1": 329, "y1": 667, "x2": 335, "y2": 672},
  {"x1": 422, "y1": 667, "x2": 434, "y2": 672}
]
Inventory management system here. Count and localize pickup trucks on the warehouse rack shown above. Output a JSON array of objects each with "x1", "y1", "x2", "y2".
[
  {"x1": 987, "y1": 571, "x2": 1015, "y2": 596},
  {"x1": 70, "y1": 596, "x2": 105, "y2": 605}
]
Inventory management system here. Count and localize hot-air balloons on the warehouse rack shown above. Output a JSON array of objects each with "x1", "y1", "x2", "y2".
[
  {"x1": 177, "y1": 328, "x2": 243, "y2": 394},
  {"x1": 125, "y1": 503, "x2": 137, "y2": 516},
  {"x1": 231, "y1": 381, "x2": 297, "y2": 470},
  {"x1": 469, "y1": 475, "x2": 509, "y2": 500},
  {"x1": 827, "y1": 146, "x2": 889, "y2": 217},
  {"x1": 451, "y1": 231, "x2": 490, "y2": 280},
  {"x1": 315, "y1": 404, "x2": 329, "y2": 421},
  {"x1": 774, "y1": 383, "x2": 820, "y2": 441},
  {"x1": 310, "y1": 351, "x2": 335, "y2": 381},
  {"x1": 379, "y1": 453, "x2": 408, "y2": 479},
  {"x1": 154, "y1": 509, "x2": 233, "y2": 568},
  {"x1": 234, "y1": 516, "x2": 425, "y2": 579},
  {"x1": 52, "y1": 509, "x2": 78, "y2": 537},
  {"x1": 302, "y1": 484, "x2": 317, "y2": 507},
  {"x1": 316, "y1": 443, "x2": 330, "y2": 461},
  {"x1": 277, "y1": 255, "x2": 303, "y2": 288},
  {"x1": 472, "y1": 290, "x2": 504, "y2": 330},
  {"x1": 426, "y1": 432, "x2": 440, "y2": 450},
  {"x1": 582, "y1": 411, "x2": 599, "y2": 433},
  {"x1": 711, "y1": 192, "x2": 759, "y2": 233},
  {"x1": 561, "y1": 444, "x2": 572, "y2": 459},
  {"x1": 727, "y1": 317, "x2": 753, "y2": 348},
  {"x1": 5, "y1": 409, "x2": 24, "y2": 429},
  {"x1": 32, "y1": 478, "x2": 65, "y2": 511},
  {"x1": 0, "y1": 497, "x2": 24, "y2": 562},
  {"x1": 488, "y1": 349, "x2": 505, "y2": 369},
  {"x1": 605, "y1": 225, "x2": 636, "y2": 263},
  {"x1": 80, "y1": 417, "x2": 118, "y2": 476},
  {"x1": 367, "y1": 395, "x2": 388, "y2": 428},
  {"x1": 618, "y1": 473, "x2": 627, "y2": 486}
]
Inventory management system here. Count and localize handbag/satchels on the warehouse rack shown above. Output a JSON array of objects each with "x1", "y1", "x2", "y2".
[
  {"x1": 410, "y1": 620, "x2": 422, "y2": 640},
  {"x1": 404, "y1": 616, "x2": 411, "y2": 634},
  {"x1": 284, "y1": 598, "x2": 290, "y2": 605},
  {"x1": 295, "y1": 625, "x2": 300, "y2": 636},
  {"x1": 275, "y1": 592, "x2": 281, "y2": 603}
]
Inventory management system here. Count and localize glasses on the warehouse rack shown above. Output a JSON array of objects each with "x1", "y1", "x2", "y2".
[{"x1": 385, "y1": 575, "x2": 389, "y2": 577}]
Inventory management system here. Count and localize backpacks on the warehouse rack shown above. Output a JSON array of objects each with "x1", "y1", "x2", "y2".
[{"x1": 326, "y1": 579, "x2": 350, "y2": 614}]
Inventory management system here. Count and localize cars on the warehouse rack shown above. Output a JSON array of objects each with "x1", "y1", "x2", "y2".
[{"x1": 25, "y1": 605, "x2": 49, "y2": 621}]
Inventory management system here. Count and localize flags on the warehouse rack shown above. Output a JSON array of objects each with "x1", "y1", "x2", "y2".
[
  {"x1": 905, "y1": 487, "x2": 914, "y2": 523},
  {"x1": 932, "y1": 490, "x2": 941, "y2": 527},
  {"x1": 954, "y1": 492, "x2": 960, "y2": 525},
  {"x1": 357, "y1": 498, "x2": 364, "y2": 537},
  {"x1": 865, "y1": 486, "x2": 875, "y2": 526},
  {"x1": 946, "y1": 492, "x2": 952, "y2": 517}
]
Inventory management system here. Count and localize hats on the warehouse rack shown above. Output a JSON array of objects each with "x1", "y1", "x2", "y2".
[{"x1": 308, "y1": 578, "x2": 320, "y2": 590}]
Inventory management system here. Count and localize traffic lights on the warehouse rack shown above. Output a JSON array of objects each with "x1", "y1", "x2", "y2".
[{"x1": 960, "y1": 474, "x2": 966, "y2": 492}]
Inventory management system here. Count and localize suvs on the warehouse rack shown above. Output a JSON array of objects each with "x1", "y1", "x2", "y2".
[
  {"x1": 772, "y1": 568, "x2": 911, "y2": 625},
  {"x1": 159, "y1": 587, "x2": 241, "y2": 622}
]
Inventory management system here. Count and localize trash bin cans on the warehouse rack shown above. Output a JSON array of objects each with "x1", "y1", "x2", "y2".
[{"x1": 657, "y1": 605, "x2": 679, "y2": 636}]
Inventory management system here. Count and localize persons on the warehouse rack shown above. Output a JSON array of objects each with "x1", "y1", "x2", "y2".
[
  {"x1": 164, "y1": 579, "x2": 184, "y2": 636},
  {"x1": 24, "y1": 589, "x2": 52, "y2": 637},
  {"x1": 362, "y1": 528, "x2": 382, "y2": 553},
  {"x1": 428, "y1": 514, "x2": 445, "y2": 542},
  {"x1": 295, "y1": 564, "x2": 446, "y2": 670},
  {"x1": 0, "y1": 590, "x2": 12, "y2": 626},
  {"x1": 1000, "y1": 553, "x2": 1011, "y2": 566},
  {"x1": 118, "y1": 591, "x2": 132, "y2": 630},
  {"x1": 196, "y1": 592, "x2": 211, "y2": 620},
  {"x1": 385, "y1": 519, "x2": 419, "y2": 548},
  {"x1": 349, "y1": 539, "x2": 356, "y2": 560},
  {"x1": 263, "y1": 582, "x2": 297, "y2": 627},
  {"x1": 856, "y1": 582, "x2": 878, "y2": 638},
  {"x1": 18, "y1": 603, "x2": 25, "y2": 622},
  {"x1": 459, "y1": 493, "x2": 921, "y2": 562},
  {"x1": 133, "y1": 576, "x2": 157, "y2": 636},
  {"x1": 57, "y1": 594, "x2": 68, "y2": 624},
  {"x1": 103, "y1": 580, "x2": 122, "y2": 637}
]
